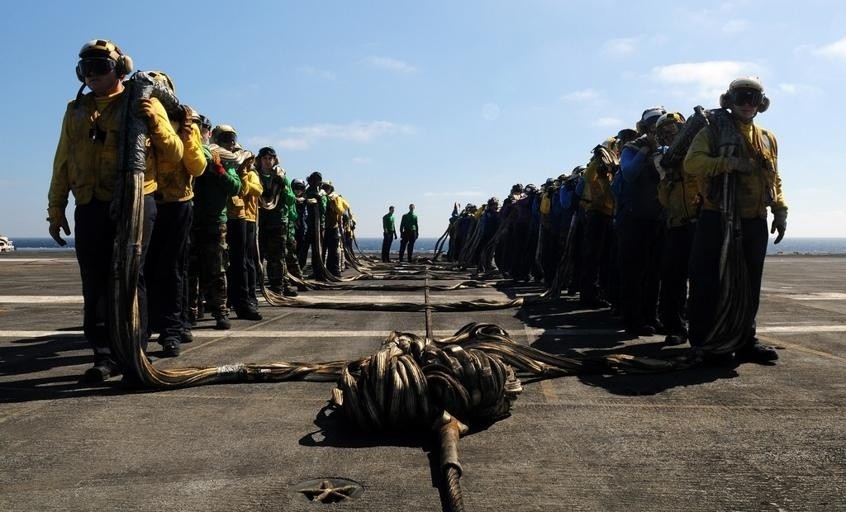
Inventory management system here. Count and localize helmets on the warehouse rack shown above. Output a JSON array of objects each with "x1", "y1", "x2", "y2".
[
  {"x1": 321, "y1": 179, "x2": 334, "y2": 195},
  {"x1": 720, "y1": 79, "x2": 769, "y2": 113},
  {"x1": 307, "y1": 171, "x2": 323, "y2": 183},
  {"x1": 635, "y1": 107, "x2": 668, "y2": 137},
  {"x1": 291, "y1": 177, "x2": 306, "y2": 191},
  {"x1": 141, "y1": 69, "x2": 177, "y2": 94},
  {"x1": 654, "y1": 111, "x2": 686, "y2": 147},
  {"x1": 601, "y1": 137, "x2": 623, "y2": 161},
  {"x1": 463, "y1": 165, "x2": 585, "y2": 214},
  {"x1": 613, "y1": 129, "x2": 639, "y2": 155}
]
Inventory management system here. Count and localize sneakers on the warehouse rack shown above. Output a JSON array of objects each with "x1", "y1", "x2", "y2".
[
  {"x1": 213, "y1": 314, "x2": 233, "y2": 331},
  {"x1": 577, "y1": 295, "x2": 663, "y2": 337},
  {"x1": 84, "y1": 363, "x2": 114, "y2": 382},
  {"x1": 272, "y1": 286, "x2": 310, "y2": 296},
  {"x1": 733, "y1": 345, "x2": 778, "y2": 364},
  {"x1": 705, "y1": 349, "x2": 742, "y2": 370},
  {"x1": 158, "y1": 325, "x2": 195, "y2": 358},
  {"x1": 664, "y1": 325, "x2": 688, "y2": 347}
]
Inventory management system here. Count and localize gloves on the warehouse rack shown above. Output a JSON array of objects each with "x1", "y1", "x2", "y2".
[
  {"x1": 769, "y1": 206, "x2": 790, "y2": 245},
  {"x1": 729, "y1": 154, "x2": 760, "y2": 182},
  {"x1": 210, "y1": 150, "x2": 226, "y2": 176},
  {"x1": 45, "y1": 212, "x2": 71, "y2": 248},
  {"x1": 136, "y1": 97, "x2": 161, "y2": 129}
]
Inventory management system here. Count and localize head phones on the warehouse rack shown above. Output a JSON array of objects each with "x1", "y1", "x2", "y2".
[
  {"x1": 636, "y1": 108, "x2": 652, "y2": 133},
  {"x1": 720, "y1": 92, "x2": 770, "y2": 113}
]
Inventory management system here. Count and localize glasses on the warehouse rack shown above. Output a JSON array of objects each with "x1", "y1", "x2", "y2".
[
  {"x1": 76, "y1": 58, "x2": 116, "y2": 77},
  {"x1": 730, "y1": 92, "x2": 762, "y2": 107}
]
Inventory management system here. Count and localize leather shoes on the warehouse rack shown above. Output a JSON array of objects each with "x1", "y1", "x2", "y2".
[{"x1": 235, "y1": 307, "x2": 264, "y2": 321}]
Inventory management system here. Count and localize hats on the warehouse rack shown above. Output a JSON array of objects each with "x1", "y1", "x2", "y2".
[
  {"x1": 191, "y1": 110, "x2": 237, "y2": 147},
  {"x1": 255, "y1": 147, "x2": 279, "y2": 167}
]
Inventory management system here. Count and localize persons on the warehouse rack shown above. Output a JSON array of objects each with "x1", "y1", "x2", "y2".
[
  {"x1": 382, "y1": 206, "x2": 397, "y2": 263},
  {"x1": 399, "y1": 204, "x2": 419, "y2": 262}
]
